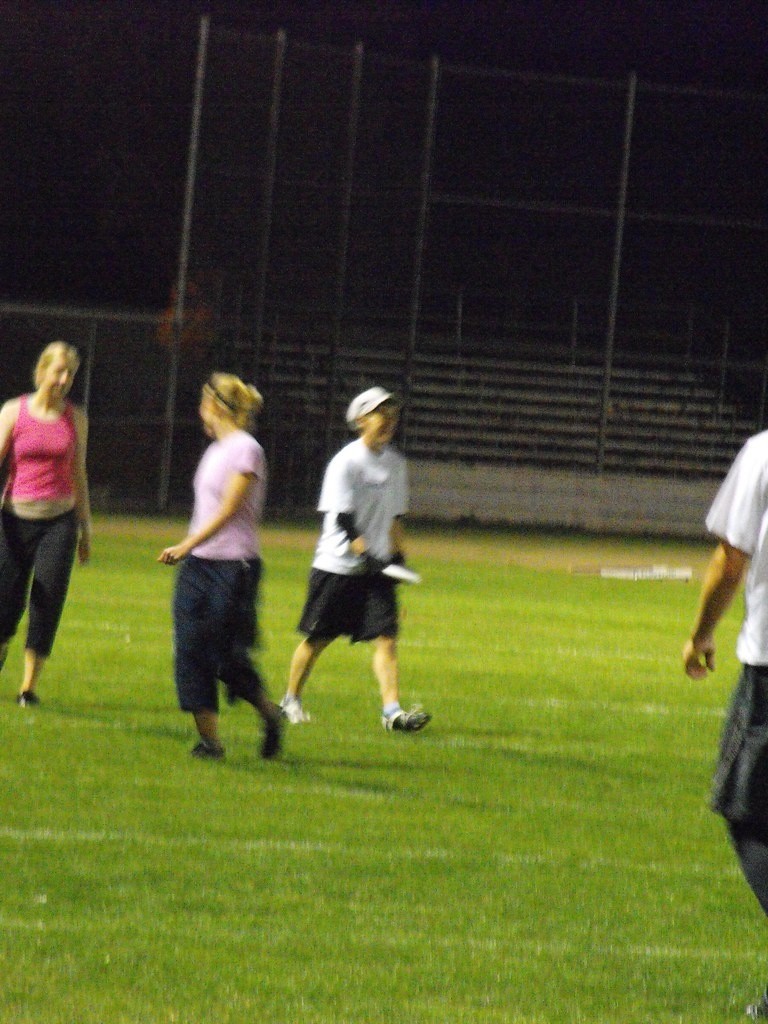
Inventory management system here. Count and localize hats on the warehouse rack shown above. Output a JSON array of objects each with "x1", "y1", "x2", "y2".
[{"x1": 345, "y1": 386, "x2": 404, "y2": 431}]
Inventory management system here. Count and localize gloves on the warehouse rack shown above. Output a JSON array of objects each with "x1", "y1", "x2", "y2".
[
  {"x1": 364, "y1": 554, "x2": 390, "y2": 575},
  {"x1": 392, "y1": 552, "x2": 406, "y2": 584}
]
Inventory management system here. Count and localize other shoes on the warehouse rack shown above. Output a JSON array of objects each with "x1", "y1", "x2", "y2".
[
  {"x1": 381, "y1": 710, "x2": 430, "y2": 732},
  {"x1": 17, "y1": 691, "x2": 39, "y2": 708},
  {"x1": 279, "y1": 699, "x2": 309, "y2": 723},
  {"x1": 259, "y1": 707, "x2": 286, "y2": 758},
  {"x1": 191, "y1": 740, "x2": 225, "y2": 764}
]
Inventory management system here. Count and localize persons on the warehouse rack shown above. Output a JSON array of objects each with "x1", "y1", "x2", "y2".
[
  {"x1": 156, "y1": 371, "x2": 286, "y2": 764},
  {"x1": 0, "y1": 341, "x2": 95, "y2": 706},
  {"x1": 680, "y1": 428, "x2": 768, "y2": 1019},
  {"x1": 279, "y1": 387, "x2": 431, "y2": 733}
]
